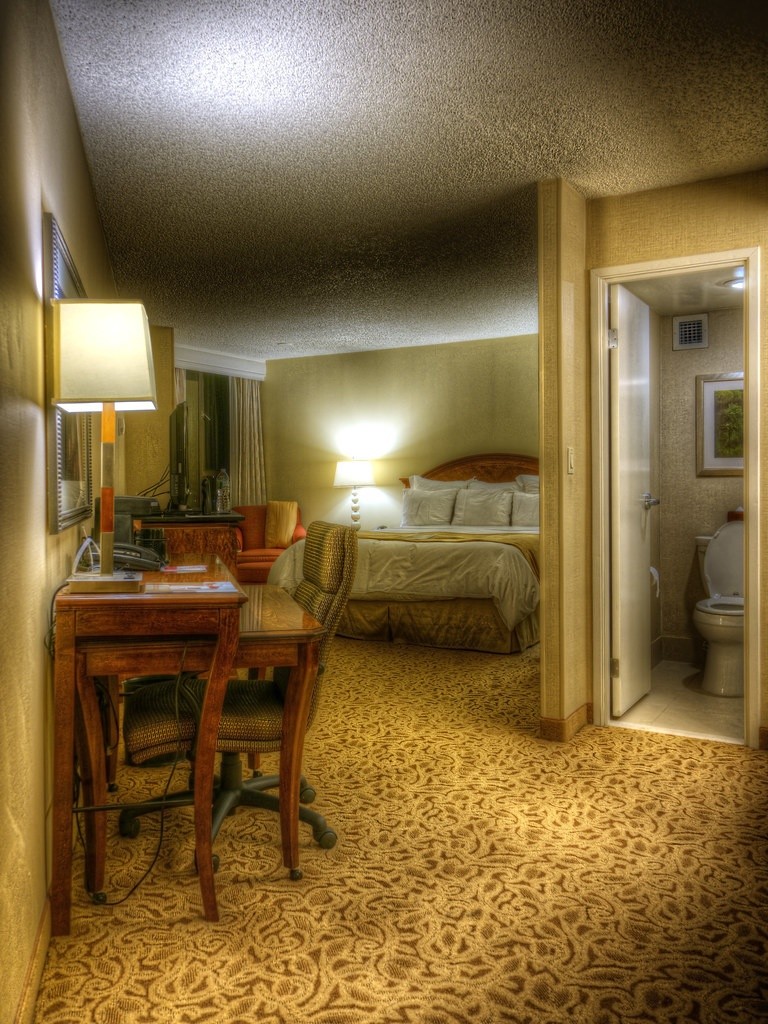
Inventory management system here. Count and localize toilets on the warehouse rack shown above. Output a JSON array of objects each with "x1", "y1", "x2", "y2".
[{"x1": 693, "y1": 520, "x2": 744, "y2": 697}]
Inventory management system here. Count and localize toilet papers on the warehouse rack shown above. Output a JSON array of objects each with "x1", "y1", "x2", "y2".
[{"x1": 649, "y1": 566, "x2": 660, "y2": 598}]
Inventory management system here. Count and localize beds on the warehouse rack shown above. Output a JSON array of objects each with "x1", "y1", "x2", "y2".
[{"x1": 267, "y1": 453, "x2": 542, "y2": 655}]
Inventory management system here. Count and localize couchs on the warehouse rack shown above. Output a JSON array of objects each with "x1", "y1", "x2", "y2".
[{"x1": 230, "y1": 505, "x2": 306, "y2": 586}]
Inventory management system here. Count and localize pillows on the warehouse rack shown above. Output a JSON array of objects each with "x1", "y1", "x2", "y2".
[
  {"x1": 400, "y1": 474, "x2": 540, "y2": 526},
  {"x1": 265, "y1": 501, "x2": 297, "y2": 549}
]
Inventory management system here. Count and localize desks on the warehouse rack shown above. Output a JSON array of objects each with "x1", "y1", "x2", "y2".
[
  {"x1": 75, "y1": 582, "x2": 326, "y2": 905},
  {"x1": 137, "y1": 521, "x2": 239, "y2": 585},
  {"x1": 144, "y1": 508, "x2": 245, "y2": 523},
  {"x1": 54, "y1": 552, "x2": 250, "y2": 936}
]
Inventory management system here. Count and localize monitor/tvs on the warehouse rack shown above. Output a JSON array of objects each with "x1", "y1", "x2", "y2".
[{"x1": 166, "y1": 401, "x2": 201, "y2": 515}]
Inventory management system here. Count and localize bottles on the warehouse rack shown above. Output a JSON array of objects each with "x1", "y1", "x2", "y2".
[{"x1": 215, "y1": 468, "x2": 231, "y2": 513}]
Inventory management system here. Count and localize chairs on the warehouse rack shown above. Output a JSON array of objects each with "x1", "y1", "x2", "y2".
[{"x1": 119, "y1": 521, "x2": 357, "y2": 875}]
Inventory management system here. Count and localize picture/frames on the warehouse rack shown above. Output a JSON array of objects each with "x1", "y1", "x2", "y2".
[
  {"x1": 696, "y1": 372, "x2": 745, "y2": 477},
  {"x1": 43, "y1": 212, "x2": 95, "y2": 535}
]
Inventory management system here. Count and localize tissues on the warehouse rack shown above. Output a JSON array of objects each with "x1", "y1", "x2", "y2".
[{"x1": 727, "y1": 506, "x2": 744, "y2": 522}]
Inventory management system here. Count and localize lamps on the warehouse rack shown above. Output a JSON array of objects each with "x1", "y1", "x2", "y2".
[
  {"x1": 50, "y1": 298, "x2": 159, "y2": 594},
  {"x1": 334, "y1": 461, "x2": 375, "y2": 532}
]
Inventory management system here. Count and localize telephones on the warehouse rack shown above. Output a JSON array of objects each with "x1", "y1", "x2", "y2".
[{"x1": 83, "y1": 542, "x2": 169, "y2": 571}]
